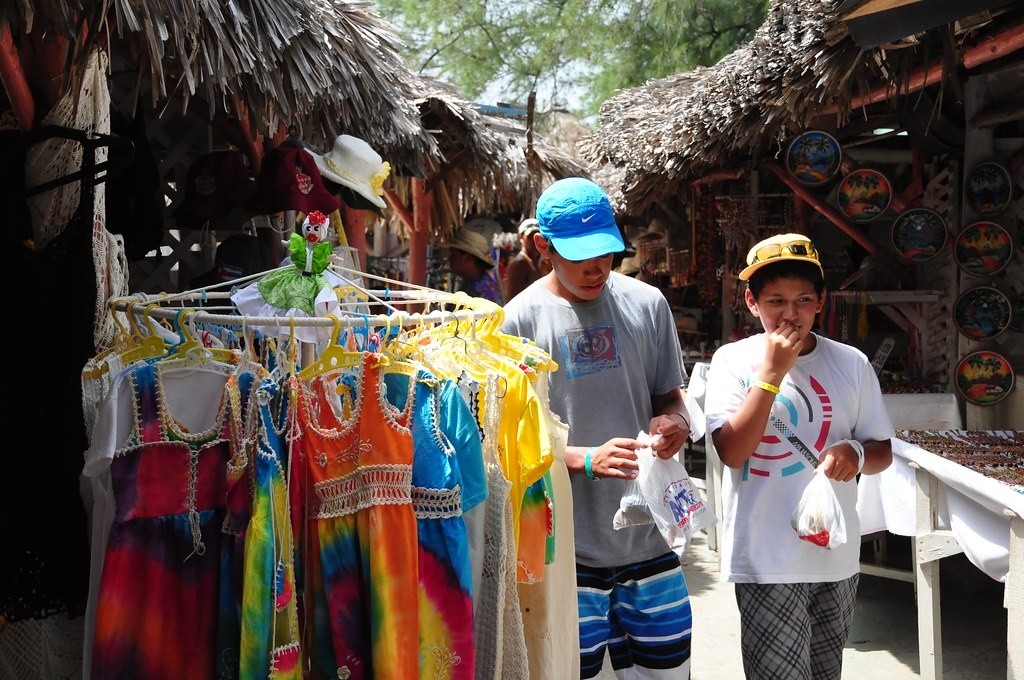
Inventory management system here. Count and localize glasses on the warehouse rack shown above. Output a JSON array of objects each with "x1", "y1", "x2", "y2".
[{"x1": 750, "y1": 240, "x2": 817, "y2": 265}]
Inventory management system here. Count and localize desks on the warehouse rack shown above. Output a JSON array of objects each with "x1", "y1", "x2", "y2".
[{"x1": 885, "y1": 428, "x2": 1024, "y2": 680}]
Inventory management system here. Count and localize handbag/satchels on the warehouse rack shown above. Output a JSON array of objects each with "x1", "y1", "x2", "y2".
[
  {"x1": 612, "y1": 470, "x2": 655, "y2": 531},
  {"x1": 634, "y1": 428, "x2": 718, "y2": 558},
  {"x1": 788, "y1": 468, "x2": 847, "y2": 550}
]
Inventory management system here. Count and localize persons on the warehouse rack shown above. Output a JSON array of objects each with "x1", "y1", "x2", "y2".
[
  {"x1": 442, "y1": 231, "x2": 501, "y2": 303},
  {"x1": 505, "y1": 218, "x2": 551, "y2": 303},
  {"x1": 694, "y1": 235, "x2": 896, "y2": 680},
  {"x1": 503, "y1": 176, "x2": 697, "y2": 680}
]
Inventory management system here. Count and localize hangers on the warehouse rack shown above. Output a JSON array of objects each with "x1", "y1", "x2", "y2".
[
  {"x1": 82, "y1": 212, "x2": 560, "y2": 395},
  {"x1": 275, "y1": 125, "x2": 307, "y2": 153},
  {"x1": 0, "y1": 104, "x2": 140, "y2": 202}
]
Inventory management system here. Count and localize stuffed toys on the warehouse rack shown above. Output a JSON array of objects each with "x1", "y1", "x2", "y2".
[{"x1": 230, "y1": 210, "x2": 344, "y2": 344}]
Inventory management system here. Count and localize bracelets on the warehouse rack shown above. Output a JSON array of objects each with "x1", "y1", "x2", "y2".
[
  {"x1": 759, "y1": 382, "x2": 779, "y2": 394},
  {"x1": 671, "y1": 412, "x2": 691, "y2": 435},
  {"x1": 584, "y1": 445, "x2": 601, "y2": 482}
]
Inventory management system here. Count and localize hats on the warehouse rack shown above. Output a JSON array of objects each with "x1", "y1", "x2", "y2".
[
  {"x1": 517, "y1": 217, "x2": 540, "y2": 237},
  {"x1": 435, "y1": 228, "x2": 496, "y2": 270},
  {"x1": 536, "y1": 176, "x2": 626, "y2": 262},
  {"x1": 738, "y1": 233, "x2": 825, "y2": 281},
  {"x1": 303, "y1": 134, "x2": 390, "y2": 209},
  {"x1": 246, "y1": 140, "x2": 340, "y2": 221},
  {"x1": 184, "y1": 149, "x2": 247, "y2": 218},
  {"x1": 196, "y1": 234, "x2": 273, "y2": 290}
]
